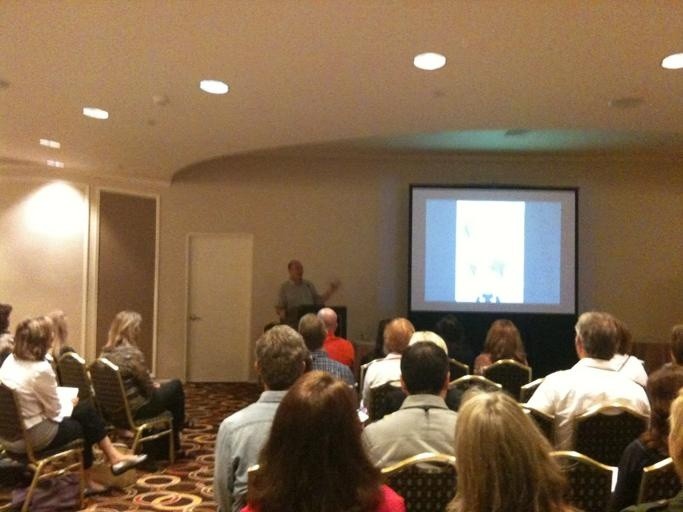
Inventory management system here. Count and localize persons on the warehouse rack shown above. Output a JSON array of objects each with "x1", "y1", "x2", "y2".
[
  {"x1": 361, "y1": 317, "x2": 415, "y2": 409},
  {"x1": 524, "y1": 311, "x2": 651, "y2": 451},
  {"x1": 318, "y1": 306, "x2": 356, "y2": 369},
  {"x1": 435, "y1": 314, "x2": 465, "y2": 361},
  {"x1": 213, "y1": 326, "x2": 309, "y2": 512},
  {"x1": 445, "y1": 392, "x2": 588, "y2": 512},
  {"x1": 363, "y1": 341, "x2": 458, "y2": 468},
  {"x1": 0, "y1": 317, "x2": 148, "y2": 496},
  {"x1": 48, "y1": 309, "x2": 97, "y2": 397},
  {"x1": 238, "y1": 369, "x2": 405, "y2": 512},
  {"x1": 619, "y1": 387, "x2": 683, "y2": 512},
  {"x1": 665, "y1": 325, "x2": 682, "y2": 363},
  {"x1": 456, "y1": 383, "x2": 516, "y2": 414},
  {"x1": 0, "y1": 301, "x2": 16, "y2": 353},
  {"x1": 275, "y1": 260, "x2": 340, "y2": 322},
  {"x1": 616, "y1": 363, "x2": 683, "y2": 505},
  {"x1": 408, "y1": 330, "x2": 450, "y2": 355},
  {"x1": 299, "y1": 313, "x2": 358, "y2": 411},
  {"x1": 472, "y1": 318, "x2": 528, "y2": 374},
  {"x1": 97, "y1": 311, "x2": 195, "y2": 456},
  {"x1": 610, "y1": 319, "x2": 648, "y2": 387}
]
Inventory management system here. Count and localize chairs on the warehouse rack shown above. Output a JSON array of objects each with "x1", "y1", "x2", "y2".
[
  {"x1": 447, "y1": 359, "x2": 470, "y2": 383},
  {"x1": 548, "y1": 450, "x2": 617, "y2": 511},
  {"x1": 58, "y1": 353, "x2": 117, "y2": 456},
  {"x1": 482, "y1": 359, "x2": 532, "y2": 401},
  {"x1": 0, "y1": 380, "x2": 84, "y2": 512},
  {"x1": 368, "y1": 380, "x2": 407, "y2": 423},
  {"x1": 573, "y1": 403, "x2": 652, "y2": 466},
  {"x1": 521, "y1": 403, "x2": 559, "y2": 446},
  {"x1": 640, "y1": 457, "x2": 681, "y2": 503},
  {"x1": 89, "y1": 359, "x2": 175, "y2": 466},
  {"x1": 443, "y1": 374, "x2": 504, "y2": 413},
  {"x1": 382, "y1": 450, "x2": 458, "y2": 512}
]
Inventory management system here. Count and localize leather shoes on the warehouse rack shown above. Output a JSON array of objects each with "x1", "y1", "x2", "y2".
[{"x1": 111, "y1": 453, "x2": 148, "y2": 475}]
